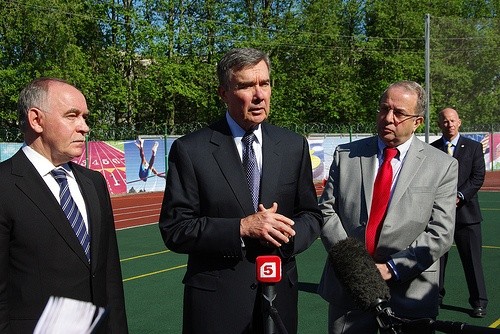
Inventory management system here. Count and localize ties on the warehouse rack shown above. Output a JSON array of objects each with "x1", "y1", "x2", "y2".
[
  {"x1": 50, "y1": 169, "x2": 90, "y2": 264},
  {"x1": 366, "y1": 147, "x2": 397, "y2": 256},
  {"x1": 447, "y1": 143, "x2": 453, "y2": 157},
  {"x1": 242, "y1": 132, "x2": 260, "y2": 214}
]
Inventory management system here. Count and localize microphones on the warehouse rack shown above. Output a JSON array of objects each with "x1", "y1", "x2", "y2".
[
  {"x1": 328, "y1": 238, "x2": 435, "y2": 334},
  {"x1": 255, "y1": 256, "x2": 288, "y2": 334}
]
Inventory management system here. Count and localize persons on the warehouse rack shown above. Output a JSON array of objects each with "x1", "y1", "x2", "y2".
[
  {"x1": 0, "y1": 77, "x2": 129, "y2": 334},
  {"x1": 138, "y1": 139, "x2": 166, "y2": 182},
  {"x1": 317, "y1": 81, "x2": 459, "y2": 334},
  {"x1": 428, "y1": 107, "x2": 487, "y2": 318},
  {"x1": 159, "y1": 49, "x2": 323, "y2": 334}
]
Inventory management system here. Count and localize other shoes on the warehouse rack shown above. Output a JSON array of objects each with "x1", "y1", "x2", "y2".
[{"x1": 473, "y1": 308, "x2": 486, "y2": 317}]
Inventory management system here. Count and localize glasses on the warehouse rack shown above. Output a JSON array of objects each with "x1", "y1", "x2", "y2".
[{"x1": 376, "y1": 105, "x2": 419, "y2": 119}]
